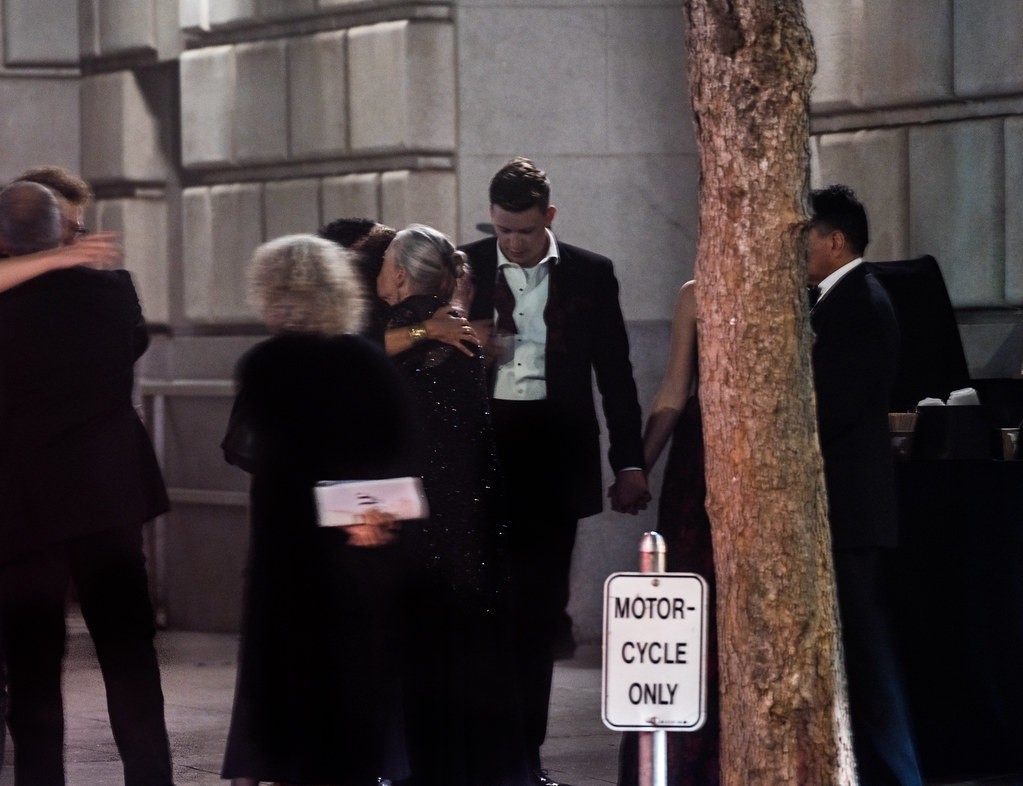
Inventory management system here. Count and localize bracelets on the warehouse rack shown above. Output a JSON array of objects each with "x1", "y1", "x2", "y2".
[{"x1": 408, "y1": 321, "x2": 428, "y2": 343}]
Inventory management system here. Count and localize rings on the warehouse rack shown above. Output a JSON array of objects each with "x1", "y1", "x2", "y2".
[
  {"x1": 464, "y1": 326, "x2": 470, "y2": 334},
  {"x1": 461, "y1": 317, "x2": 466, "y2": 325}
]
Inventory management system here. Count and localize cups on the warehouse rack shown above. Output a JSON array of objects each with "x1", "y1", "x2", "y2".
[{"x1": 1000, "y1": 428, "x2": 1021, "y2": 460}]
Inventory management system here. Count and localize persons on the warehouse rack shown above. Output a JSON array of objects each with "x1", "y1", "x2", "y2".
[
  {"x1": 607, "y1": 277, "x2": 724, "y2": 786},
  {"x1": 220, "y1": 218, "x2": 515, "y2": 786},
  {"x1": 802, "y1": 187, "x2": 926, "y2": 786},
  {"x1": 455, "y1": 163, "x2": 654, "y2": 786},
  {"x1": 0, "y1": 168, "x2": 176, "y2": 786}
]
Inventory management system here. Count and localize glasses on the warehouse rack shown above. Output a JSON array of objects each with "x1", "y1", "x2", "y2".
[{"x1": 69, "y1": 227, "x2": 89, "y2": 240}]
